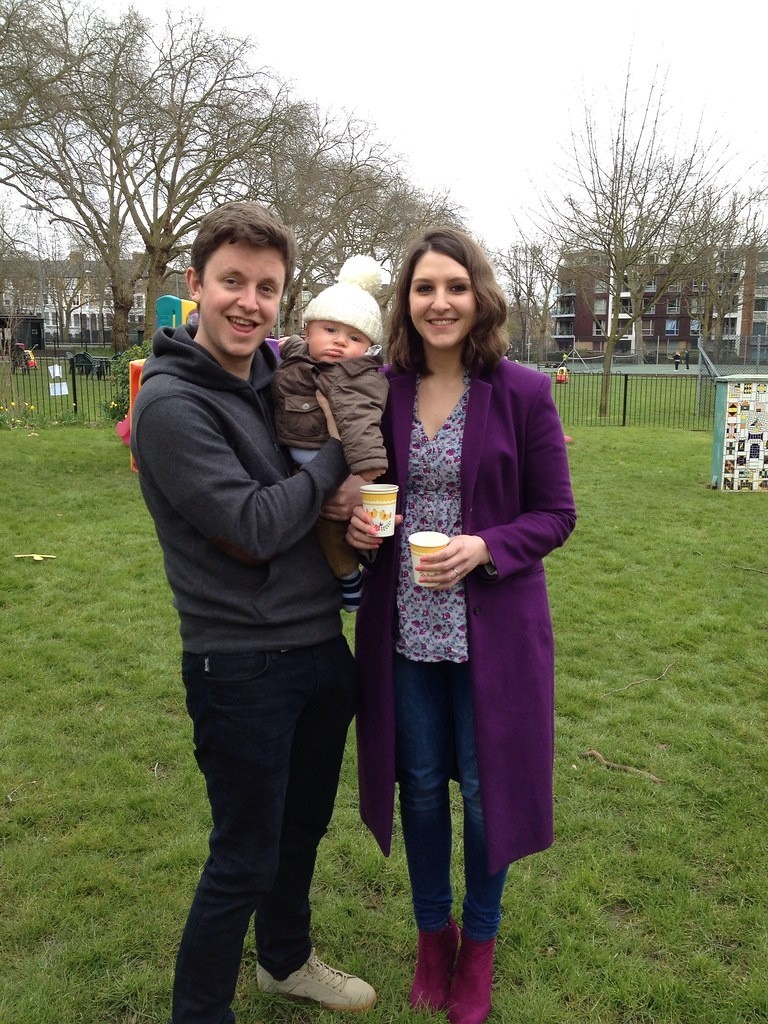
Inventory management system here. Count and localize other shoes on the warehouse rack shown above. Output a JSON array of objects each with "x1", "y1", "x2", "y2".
[
  {"x1": 685, "y1": 369, "x2": 690, "y2": 371},
  {"x1": 674, "y1": 370, "x2": 679, "y2": 372}
]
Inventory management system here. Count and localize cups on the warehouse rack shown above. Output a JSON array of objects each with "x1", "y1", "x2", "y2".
[
  {"x1": 408, "y1": 531, "x2": 450, "y2": 588},
  {"x1": 359, "y1": 484, "x2": 399, "y2": 538}
]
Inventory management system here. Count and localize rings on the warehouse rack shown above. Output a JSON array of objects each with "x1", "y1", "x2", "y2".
[{"x1": 453, "y1": 567, "x2": 459, "y2": 577}]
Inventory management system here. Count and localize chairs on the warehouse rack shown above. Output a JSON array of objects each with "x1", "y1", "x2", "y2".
[{"x1": 66, "y1": 351, "x2": 112, "y2": 381}]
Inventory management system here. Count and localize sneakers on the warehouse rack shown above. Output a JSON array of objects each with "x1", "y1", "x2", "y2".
[{"x1": 255, "y1": 946, "x2": 378, "y2": 1014}]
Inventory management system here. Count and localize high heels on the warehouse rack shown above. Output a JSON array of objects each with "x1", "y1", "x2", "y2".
[
  {"x1": 410, "y1": 911, "x2": 460, "y2": 1013},
  {"x1": 446, "y1": 926, "x2": 496, "y2": 1024}
]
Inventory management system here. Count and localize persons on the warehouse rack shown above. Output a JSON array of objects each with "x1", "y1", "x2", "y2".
[
  {"x1": 273, "y1": 255, "x2": 387, "y2": 612},
  {"x1": 562, "y1": 352, "x2": 568, "y2": 364},
  {"x1": 672, "y1": 349, "x2": 691, "y2": 372},
  {"x1": 355, "y1": 229, "x2": 576, "y2": 1023},
  {"x1": 129, "y1": 203, "x2": 378, "y2": 1024}
]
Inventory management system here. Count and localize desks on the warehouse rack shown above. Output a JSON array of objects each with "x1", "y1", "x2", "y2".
[{"x1": 92, "y1": 357, "x2": 112, "y2": 374}]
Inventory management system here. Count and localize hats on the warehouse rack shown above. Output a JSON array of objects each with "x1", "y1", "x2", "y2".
[{"x1": 301, "y1": 252, "x2": 384, "y2": 347}]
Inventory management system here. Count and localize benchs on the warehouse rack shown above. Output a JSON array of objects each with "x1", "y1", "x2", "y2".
[{"x1": 537, "y1": 362, "x2": 565, "y2": 371}]
